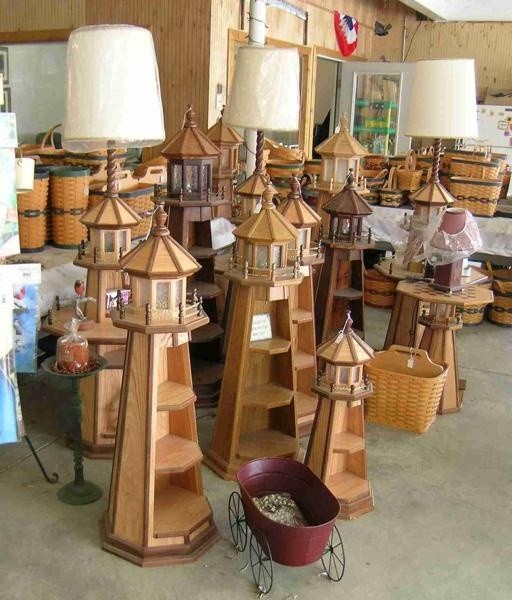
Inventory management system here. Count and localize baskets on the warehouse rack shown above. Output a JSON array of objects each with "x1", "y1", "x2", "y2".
[
  {"x1": 362, "y1": 263, "x2": 512, "y2": 330},
  {"x1": 362, "y1": 343, "x2": 450, "y2": 439},
  {"x1": 90, "y1": 182, "x2": 167, "y2": 243},
  {"x1": 19, "y1": 164, "x2": 51, "y2": 255},
  {"x1": 15, "y1": 124, "x2": 64, "y2": 163},
  {"x1": 64, "y1": 148, "x2": 129, "y2": 194},
  {"x1": 259, "y1": 134, "x2": 330, "y2": 195},
  {"x1": 50, "y1": 164, "x2": 90, "y2": 251}
]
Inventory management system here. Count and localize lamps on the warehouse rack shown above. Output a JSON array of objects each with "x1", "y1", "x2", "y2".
[
  {"x1": 373, "y1": 58, "x2": 489, "y2": 352},
  {"x1": 41, "y1": 23, "x2": 167, "y2": 461},
  {"x1": 222, "y1": 44, "x2": 302, "y2": 227}
]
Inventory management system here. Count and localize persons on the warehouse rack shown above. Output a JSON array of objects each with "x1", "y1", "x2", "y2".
[{"x1": 73, "y1": 280, "x2": 97, "y2": 331}]
